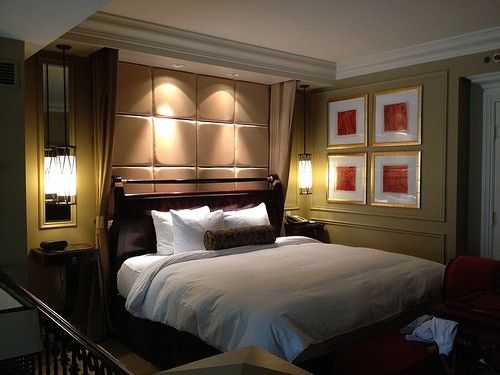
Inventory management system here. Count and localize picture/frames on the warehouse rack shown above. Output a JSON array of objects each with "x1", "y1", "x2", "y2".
[
  {"x1": 324, "y1": 92, "x2": 370, "y2": 149},
  {"x1": 324, "y1": 151, "x2": 368, "y2": 206},
  {"x1": 370, "y1": 84, "x2": 424, "y2": 148},
  {"x1": 370, "y1": 150, "x2": 422, "y2": 210}
]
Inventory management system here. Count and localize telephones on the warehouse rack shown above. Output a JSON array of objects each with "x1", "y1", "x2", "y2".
[{"x1": 284, "y1": 215, "x2": 309, "y2": 224}]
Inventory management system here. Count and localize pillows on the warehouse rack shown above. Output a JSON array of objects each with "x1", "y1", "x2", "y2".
[
  {"x1": 214, "y1": 203, "x2": 256, "y2": 216},
  {"x1": 203, "y1": 224, "x2": 277, "y2": 251},
  {"x1": 217, "y1": 202, "x2": 270, "y2": 229},
  {"x1": 151, "y1": 205, "x2": 210, "y2": 256},
  {"x1": 168, "y1": 207, "x2": 224, "y2": 256}
]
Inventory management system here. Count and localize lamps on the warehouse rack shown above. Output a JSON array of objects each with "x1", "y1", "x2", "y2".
[
  {"x1": 43, "y1": 63, "x2": 57, "y2": 204},
  {"x1": 53, "y1": 44, "x2": 79, "y2": 207},
  {"x1": 296, "y1": 84, "x2": 318, "y2": 196}
]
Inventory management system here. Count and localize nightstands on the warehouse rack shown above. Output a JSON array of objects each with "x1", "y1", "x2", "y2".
[
  {"x1": 26, "y1": 245, "x2": 100, "y2": 349},
  {"x1": 283, "y1": 220, "x2": 325, "y2": 242}
]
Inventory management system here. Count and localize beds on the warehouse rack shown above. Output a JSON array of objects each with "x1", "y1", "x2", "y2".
[{"x1": 106, "y1": 172, "x2": 446, "y2": 367}]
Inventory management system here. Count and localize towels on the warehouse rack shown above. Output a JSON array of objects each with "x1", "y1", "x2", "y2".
[{"x1": 400, "y1": 314, "x2": 460, "y2": 356}]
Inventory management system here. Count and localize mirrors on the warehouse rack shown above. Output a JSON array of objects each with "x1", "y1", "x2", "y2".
[{"x1": 38, "y1": 57, "x2": 80, "y2": 231}]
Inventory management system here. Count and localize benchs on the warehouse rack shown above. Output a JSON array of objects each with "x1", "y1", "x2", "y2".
[{"x1": 331, "y1": 254, "x2": 500, "y2": 375}]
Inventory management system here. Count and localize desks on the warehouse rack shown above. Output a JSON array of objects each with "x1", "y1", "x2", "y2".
[{"x1": 429, "y1": 286, "x2": 500, "y2": 375}]
